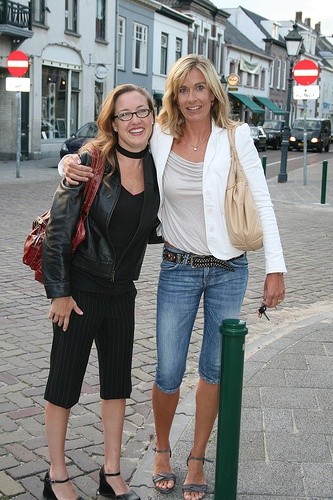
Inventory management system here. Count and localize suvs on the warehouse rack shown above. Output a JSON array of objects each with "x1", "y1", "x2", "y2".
[{"x1": 257, "y1": 120, "x2": 289, "y2": 150}]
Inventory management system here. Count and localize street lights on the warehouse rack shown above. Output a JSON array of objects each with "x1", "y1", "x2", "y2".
[{"x1": 277, "y1": 22, "x2": 304, "y2": 184}]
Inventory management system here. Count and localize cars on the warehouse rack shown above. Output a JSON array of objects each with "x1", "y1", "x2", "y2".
[
  {"x1": 249, "y1": 126, "x2": 268, "y2": 152},
  {"x1": 59, "y1": 121, "x2": 99, "y2": 158}
]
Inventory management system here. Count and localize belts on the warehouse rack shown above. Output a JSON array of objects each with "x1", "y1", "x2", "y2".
[{"x1": 163, "y1": 249, "x2": 244, "y2": 272}]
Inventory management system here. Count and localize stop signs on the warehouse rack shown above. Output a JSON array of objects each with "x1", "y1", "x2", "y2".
[
  {"x1": 7, "y1": 51, "x2": 29, "y2": 76},
  {"x1": 292, "y1": 61, "x2": 318, "y2": 85}
]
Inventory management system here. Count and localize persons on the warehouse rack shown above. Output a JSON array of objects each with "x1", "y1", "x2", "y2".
[
  {"x1": 56, "y1": 55, "x2": 288, "y2": 500},
  {"x1": 40, "y1": 83, "x2": 160, "y2": 500}
]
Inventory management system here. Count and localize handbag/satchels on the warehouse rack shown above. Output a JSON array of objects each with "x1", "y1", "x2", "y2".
[
  {"x1": 22, "y1": 140, "x2": 106, "y2": 281},
  {"x1": 225, "y1": 123, "x2": 264, "y2": 251}
]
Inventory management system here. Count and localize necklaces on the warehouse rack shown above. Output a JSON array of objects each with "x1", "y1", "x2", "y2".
[
  {"x1": 186, "y1": 130, "x2": 210, "y2": 151},
  {"x1": 116, "y1": 144, "x2": 150, "y2": 159}
]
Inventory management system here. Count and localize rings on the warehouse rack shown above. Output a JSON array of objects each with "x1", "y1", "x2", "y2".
[{"x1": 278, "y1": 299, "x2": 283, "y2": 302}]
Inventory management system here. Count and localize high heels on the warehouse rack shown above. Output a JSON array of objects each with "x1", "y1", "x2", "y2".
[
  {"x1": 40, "y1": 470, "x2": 85, "y2": 500},
  {"x1": 152, "y1": 445, "x2": 177, "y2": 493},
  {"x1": 181, "y1": 451, "x2": 214, "y2": 500},
  {"x1": 98, "y1": 465, "x2": 142, "y2": 500}
]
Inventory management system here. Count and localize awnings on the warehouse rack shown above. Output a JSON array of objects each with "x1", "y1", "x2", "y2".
[
  {"x1": 254, "y1": 96, "x2": 287, "y2": 115},
  {"x1": 230, "y1": 93, "x2": 266, "y2": 113}
]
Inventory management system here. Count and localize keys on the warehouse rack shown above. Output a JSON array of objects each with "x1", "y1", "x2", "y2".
[{"x1": 259, "y1": 306, "x2": 270, "y2": 321}]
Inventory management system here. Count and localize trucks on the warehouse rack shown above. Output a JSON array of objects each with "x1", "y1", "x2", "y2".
[{"x1": 289, "y1": 117, "x2": 332, "y2": 152}]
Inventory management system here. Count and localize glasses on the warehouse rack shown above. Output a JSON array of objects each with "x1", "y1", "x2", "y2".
[{"x1": 113, "y1": 108, "x2": 152, "y2": 121}]
